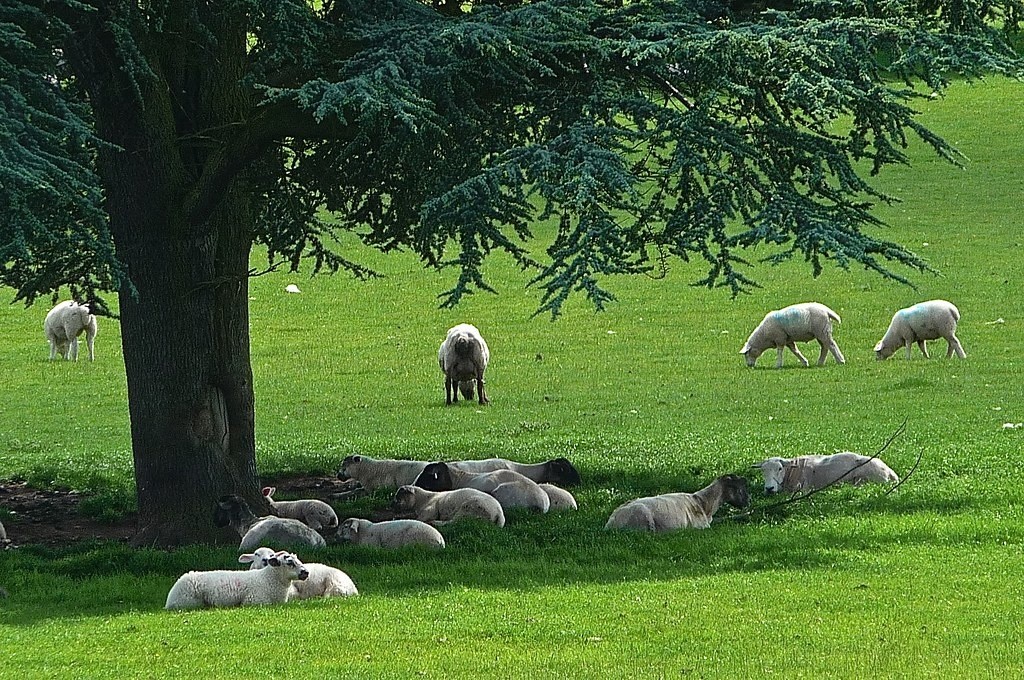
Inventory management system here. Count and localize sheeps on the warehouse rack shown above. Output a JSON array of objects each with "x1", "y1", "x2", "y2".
[
  {"x1": 392, "y1": 487, "x2": 506, "y2": 528},
  {"x1": 751, "y1": 452, "x2": 899, "y2": 494},
  {"x1": 536, "y1": 483, "x2": 578, "y2": 511},
  {"x1": 42, "y1": 297, "x2": 98, "y2": 362},
  {"x1": 437, "y1": 319, "x2": 491, "y2": 406},
  {"x1": 601, "y1": 473, "x2": 752, "y2": 530},
  {"x1": 413, "y1": 462, "x2": 550, "y2": 513},
  {"x1": 873, "y1": 298, "x2": 969, "y2": 361},
  {"x1": 336, "y1": 454, "x2": 585, "y2": 493},
  {"x1": 332, "y1": 518, "x2": 446, "y2": 549},
  {"x1": 237, "y1": 546, "x2": 359, "y2": 609},
  {"x1": 163, "y1": 547, "x2": 311, "y2": 612},
  {"x1": 261, "y1": 487, "x2": 338, "y2": 529},
  {"x1": 216, "y1": 495, "x2": 327, "y2": 547},
  {"x1": 737, "y1": 302, "x2": 845, "y2": 368}
]
